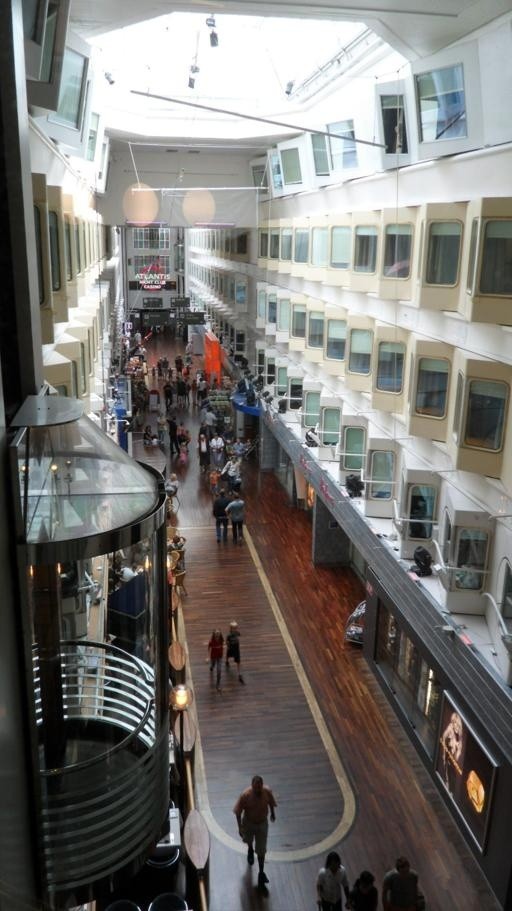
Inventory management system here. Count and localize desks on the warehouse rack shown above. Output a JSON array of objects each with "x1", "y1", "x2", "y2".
[{"x1": 167, "y1": 588, "x2": 210, "y2": 871}]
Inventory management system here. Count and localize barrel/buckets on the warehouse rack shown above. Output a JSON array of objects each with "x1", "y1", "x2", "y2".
[
  {"x1": 104, "y1": 899, "x2": 141, "y2": 911},
  {"x1": 148, "y1": 893, "x2": 190, "y2": 911},
  {"x1": 144, "y1": 844, "x2": 189, "y2": 898}
]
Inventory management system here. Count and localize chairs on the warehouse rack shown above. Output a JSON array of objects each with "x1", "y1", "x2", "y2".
[{"x1": 166, "y1": 477, "x2": 187, "y2": 595}]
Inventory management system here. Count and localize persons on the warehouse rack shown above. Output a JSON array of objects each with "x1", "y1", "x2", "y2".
[
  {"x1": 206, "y1": 628, "x2": 228, "y2": 695},
  {"x1": 345, "y1": 871, "x2": 379, "y2": 911},
  {"x1": 382, "y1": 857, "x2": 424, "y2": 911},
  {"x1": 314, "y1": 852, "x2": 350, "y2": 911},
  {"x1": 226, "y1": 621, "x2": 243, "y2": 683},
  {"x1": 232, "y1": 775, "x2": 277, "y2": 884},
  {"x1": 108, "y1": 339, "x2": 253, "y2": 594}
]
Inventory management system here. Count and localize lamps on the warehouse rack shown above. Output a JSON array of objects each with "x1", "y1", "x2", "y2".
[
  {"x1": 285, "y1": 80, "x2": 294, "y2": 96},
  {"x1": 109, "y1": 419, "x2": 131, "y2": 426},
  {"x1": 105, "y1": 72, "x2": 115, "y2": 85},
  {"x1": 188, "y1": 13, "x2": 219, "y2": 89}
]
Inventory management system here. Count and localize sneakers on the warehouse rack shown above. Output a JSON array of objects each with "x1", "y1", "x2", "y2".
[
  {"x1": 247, "y1": 848, "x2": 255, "y2": 865},
  {"x1": 238, "y1": 677, "x2": 245, "y2": 684},
  {"x1": 216, "y1": 685, "x2": 222, "y2": 691},
  {"x1": 233, "y1": 536, "x2": 243, "y2": 546},
  {"x1": 258, "y1": 870, "x2": 268, "y2": 883},
  {"x1": 209, "y1": 665, "x2": 214, "y2": 672},
  {"x1": 225, "y1": 660, "x2": 230, "y2": 667},
  {"x1": 217, "y1": 539, "x2": 227, "y2": 542}
]
